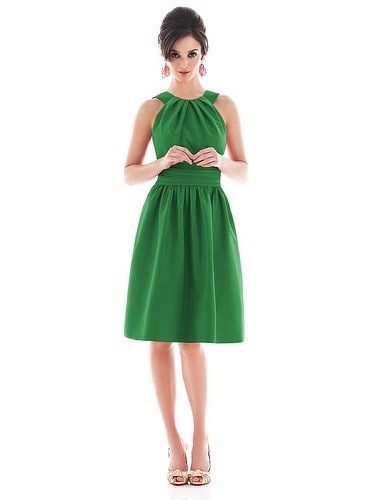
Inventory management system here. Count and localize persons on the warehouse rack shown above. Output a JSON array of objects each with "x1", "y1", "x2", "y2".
[{"x1": 118, "y1": 7, "x2": 250, "y2": 488}]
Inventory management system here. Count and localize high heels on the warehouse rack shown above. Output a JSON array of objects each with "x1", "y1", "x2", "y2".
[
  {"x1": 189, "y1": 440, "x2": 212, "y2": 486},
  {"x1": 167, "y1": 439, "x2": 189, "y2": 485}
]
